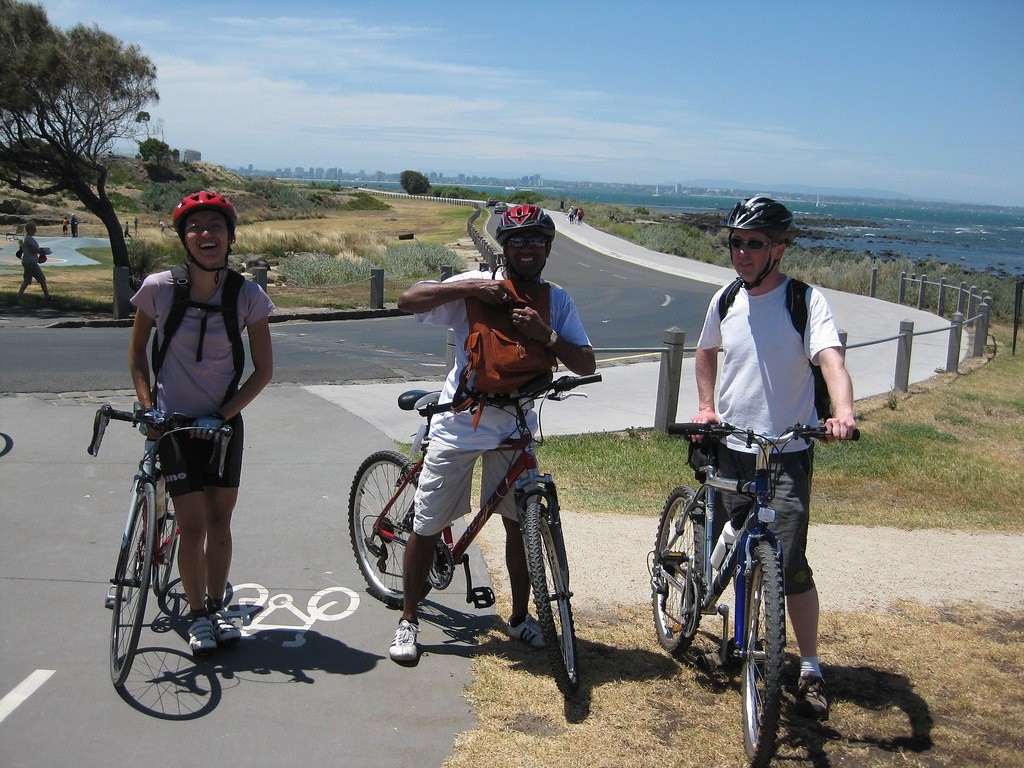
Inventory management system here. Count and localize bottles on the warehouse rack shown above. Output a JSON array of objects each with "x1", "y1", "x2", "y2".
[
  {"x1": 153, "y1": 471, "x2": 166, "y2": 520},
  {"x1": 710, "y1": 514, "x2": 744, "y2": 570}
]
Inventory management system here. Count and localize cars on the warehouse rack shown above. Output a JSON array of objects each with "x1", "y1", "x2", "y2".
[
  {"x1": 486, "y1": 198, "x2": 499, "y2": 207},
  {"x1": 494, "y1": 202, "x2": 508, "y2": 213}
]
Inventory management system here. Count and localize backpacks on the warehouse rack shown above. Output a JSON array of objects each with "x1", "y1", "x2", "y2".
[
  {"x1": 720, "y1": 275, "x2": 831, "y2": 421},
  {"x1": 152, "y1": 262, "x2": 244, "y2": 385}
]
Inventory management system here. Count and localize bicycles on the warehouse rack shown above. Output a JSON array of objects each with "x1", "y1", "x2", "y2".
[
  {"x1": 87, "y1": 401, "x2": 235, "y2": 687},
  {"x1": 347, "y1": 372, "x2": 602, "y2": 694},
  {"x1": 648, "y1": 424, "x2": 861, "y2": 766}
]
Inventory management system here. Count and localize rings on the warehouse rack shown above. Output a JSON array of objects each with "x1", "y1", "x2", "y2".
[
  {"x1": 518, "y1": 314, "x2": 523, "y2": 321},
  {"x1": 502, "y1": 293, "x2": 507, "y2": 300}
]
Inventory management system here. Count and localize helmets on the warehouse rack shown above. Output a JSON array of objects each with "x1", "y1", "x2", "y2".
[
  {"x1": 495, "y1": 205, "x2": 556, "y2": 248},
  {"x1": 172, "y1": 189, "x2": 236, "y2": 239},
  {"x1": 718, "y1": 197, "x2": 802, "y2": 248}
]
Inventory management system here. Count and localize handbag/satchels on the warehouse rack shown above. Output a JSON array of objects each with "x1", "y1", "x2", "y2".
[{"x1": 452, "y1": 278, "x2": 558, "y2": 432}]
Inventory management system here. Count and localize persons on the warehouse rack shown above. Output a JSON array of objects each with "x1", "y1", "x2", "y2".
[
  {"x1": 133, "y1": 217, "x2": 140, "y2": 231},
  {"x1": 62, "y1": 213, "x2": 78, "y2": 237},
  {"x1": 157, "y1": 219, "x2": 165, "y2": 232},
  {"x1": 124, "y1": 221, "x2": 132, "y2": 238},
  {"x1": 567, "y1": 205, "x2": 584, "y2": 225},
  {"x1": 388, "y1": 204, "x2": 597, "y2": 663},
  {"x1": 129, "y1": 191, "x2": 274, "y2": 655},
  {"x1": 17, "y1": 222, "x2": 58, "y2": 303},
  {"x1": 689, "y1": 196, "x2": 857, "y2": 720}
]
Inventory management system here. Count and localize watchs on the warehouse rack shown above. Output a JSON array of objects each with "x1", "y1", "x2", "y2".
[{"x1": 544, "y1": 329, "x2": 558, "y2": 347}]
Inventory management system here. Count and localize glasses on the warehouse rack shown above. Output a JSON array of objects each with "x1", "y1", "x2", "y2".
[
  {"x1": 182, "y1": 222, "x2": 229, "y2": 234},
  {"x1": 727, "y1": 236, "x2": 778, "y2": 249},
  {"x1": 504, "y1": 236, "x2": 551, "y2": 248}
]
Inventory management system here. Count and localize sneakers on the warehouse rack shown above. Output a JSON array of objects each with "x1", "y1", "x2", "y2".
[
  {"x1": 795, "y1": 672, "x2": 829, "y2": 717},
  {"x1": 389, "y1": 620, "x2": 421, "y2": 661},
  {"x1": 187, "y1": 617, "x2": 217, "y2": 652},
  {"x1": 507, "y1": 613, "x2": 543, "y2": 647},
  {"x1": 210, "y1": 609, "x2": 241, "y2": 641},
  {"x1": 697, "y1": 637, "x2": 765, "y2": 670}
]
